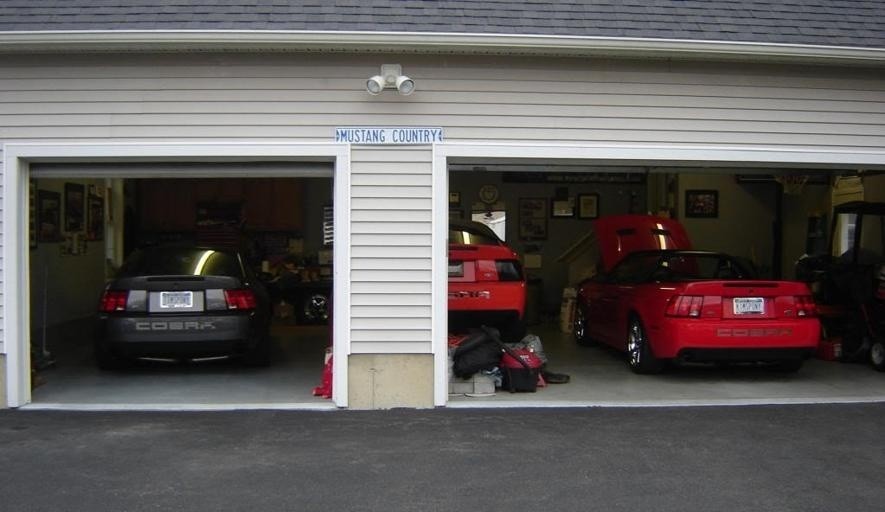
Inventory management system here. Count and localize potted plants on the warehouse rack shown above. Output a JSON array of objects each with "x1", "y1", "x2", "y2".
[
  {"x1": 576, "y1": 192, "x2": 599, "y2": 219},
  {"x1": 29, "y1": 177, "x2": 104, "y2": 250},
  {"x1": 684, "y1": 188, "x2": 720, "y2": 219},
  {"x1": 517, "y1": 196, "x2": 549, "y2": 242},
  {"x1": 551, "y1": 199, "x2": 575, "y2": 219}
]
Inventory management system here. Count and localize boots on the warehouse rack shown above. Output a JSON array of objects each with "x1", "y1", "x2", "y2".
[{"x1": 795, "y1": 201, "x2": 885, "y2": 369}]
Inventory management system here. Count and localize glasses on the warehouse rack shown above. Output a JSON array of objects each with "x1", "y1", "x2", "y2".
[{"x1": 365, "y1": 63, "x2": 416, "y2": 97}]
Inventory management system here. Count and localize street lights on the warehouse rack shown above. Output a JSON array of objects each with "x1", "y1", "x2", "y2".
[{"x1": 453, "y1": 325, "x2": 501, "y2": 380}]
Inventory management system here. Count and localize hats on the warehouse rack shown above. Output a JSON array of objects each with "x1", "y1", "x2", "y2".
[
  {"x1": 572, "y1": 212, "x2": 821, "y2": 371},
  {"x1": 100, "y1": 238, "x2": 282, "y2": 366},
  {"x1": 448, "y1": 215, "x2": 526, "y2": 331}
]
